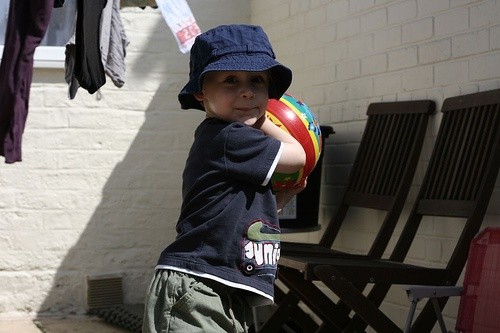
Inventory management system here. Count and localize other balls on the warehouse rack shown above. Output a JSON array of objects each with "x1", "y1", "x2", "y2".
[{"x1": 263, "y1": 92, "x2": 323, "y2": 192}]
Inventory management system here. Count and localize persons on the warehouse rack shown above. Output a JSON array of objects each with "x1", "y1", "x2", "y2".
[{"x1": 142, "y1": 24, "x2": 309, "y2": 333}]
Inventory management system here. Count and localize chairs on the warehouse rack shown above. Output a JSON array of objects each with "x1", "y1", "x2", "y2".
[
  {"x1": 403, "y1": 228, "x2": 500, "y2": 333},
  {"x1": 276, "y1": 89, "x2": 500, "y2": 333},
  {"x1": 254, "y1": 99, "x2": 435, "y2": 333}
]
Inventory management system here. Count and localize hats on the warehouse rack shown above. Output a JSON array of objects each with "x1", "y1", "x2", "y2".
[{"x1": 178, "y1": 23, "x2": 292, "y2": 111}]
